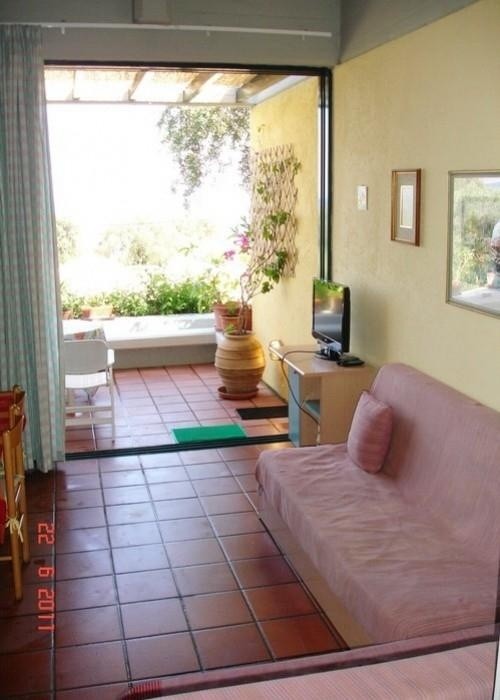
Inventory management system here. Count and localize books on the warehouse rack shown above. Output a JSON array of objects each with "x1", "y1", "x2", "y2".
[{"x1": 304, "y1": 398, "x2": 320, "y2": 419}]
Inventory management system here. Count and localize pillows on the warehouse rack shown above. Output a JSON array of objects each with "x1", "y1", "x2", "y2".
[{"x1": 348, "y1": 391, "x2": 395, "y2": 473}]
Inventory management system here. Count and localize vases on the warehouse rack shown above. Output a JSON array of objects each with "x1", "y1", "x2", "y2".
[{"x1": 211, "y1": 299, "x2": 252, "y2": 338}]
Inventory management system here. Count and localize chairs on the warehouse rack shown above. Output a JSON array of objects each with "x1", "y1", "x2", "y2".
[
  {"x1": 59, "y1": 328, "x2": 115, "y2": 448},
  {"x1": 1, "y1": 384, "x2": 32, "y2": 609}
]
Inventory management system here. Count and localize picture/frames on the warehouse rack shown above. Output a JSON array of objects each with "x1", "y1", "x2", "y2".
[{"x1": 386, "y1": 168, "x2": 500, "y2": 323}]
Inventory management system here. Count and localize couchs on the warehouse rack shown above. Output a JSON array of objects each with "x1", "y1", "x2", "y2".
[
  {"x1": 254, "y1": 361, "x2": 499, "y2": 650},
  {"x1": 136, "y1": 618, "x2": 496, "y2": 700}
]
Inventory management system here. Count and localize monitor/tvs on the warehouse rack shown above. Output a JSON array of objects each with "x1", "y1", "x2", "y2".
[{"x1": 312, "y1": 278, "x2": 350, "y2": 360}]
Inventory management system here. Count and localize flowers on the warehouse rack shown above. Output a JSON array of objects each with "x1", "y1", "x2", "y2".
[{"x1": 161, "y1": 90, "x2": 304, "y2": 305}]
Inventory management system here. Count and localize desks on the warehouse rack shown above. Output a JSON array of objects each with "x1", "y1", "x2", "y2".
[{"x1": 272, "y1": 344, "x2": 374, "y2": 445}]
